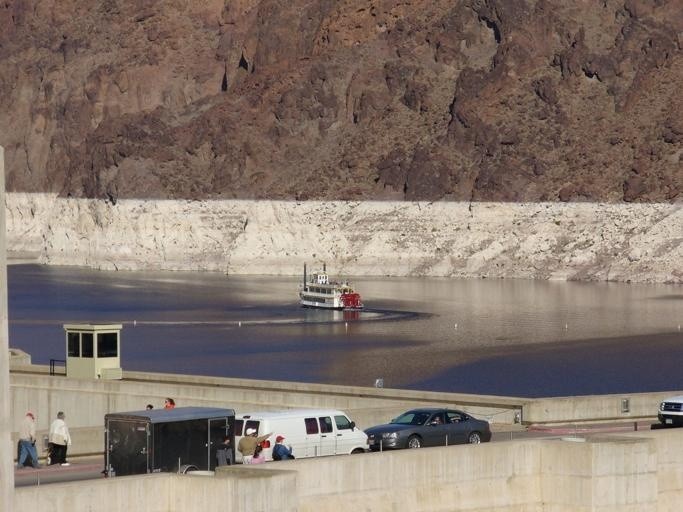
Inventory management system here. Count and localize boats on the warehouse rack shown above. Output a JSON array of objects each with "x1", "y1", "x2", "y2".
[
  {"x1": 304, "y1": 308, "x2": 385, "y2": 323},
  {"x1": 300, "y1": 263, "x2": 364, "y2": 308}
]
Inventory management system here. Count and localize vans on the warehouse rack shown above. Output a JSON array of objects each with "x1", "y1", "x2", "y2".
[{"x1": 235, "y1": 409, "x2": 371, "y2": 463}]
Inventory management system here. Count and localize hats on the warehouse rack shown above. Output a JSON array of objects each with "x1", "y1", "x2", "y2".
[
  {"x1": 276, "y1": 436, "x2": 285, "y2": 442},
  {"x1": 246, "y1": 428, "x2": 257, "y2": 436}
]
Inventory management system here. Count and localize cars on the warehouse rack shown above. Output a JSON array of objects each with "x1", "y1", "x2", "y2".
[
  {"x1": 658, "y1": 397, "x2": 683, "y2": 427},
  {"x1": 364, "y1": 408, "x2": 491, "y2": 449}
]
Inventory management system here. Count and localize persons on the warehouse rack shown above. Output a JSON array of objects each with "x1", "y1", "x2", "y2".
[
  {"x1": 17, "y1": 411, "x2": 71, "y2": 469},
  {"x1": 145, "y1": 396, "x2": 176, "y2": 410},
  {"x1": 215, "y1": 427, "x2": 294, "y2": 467}
]
